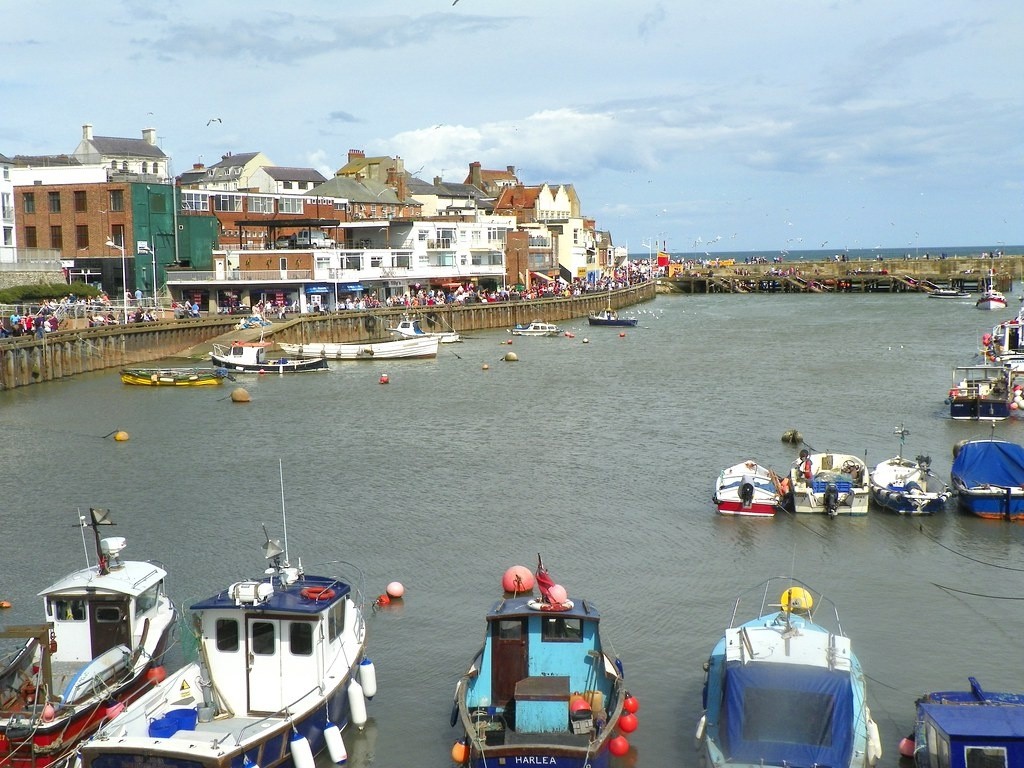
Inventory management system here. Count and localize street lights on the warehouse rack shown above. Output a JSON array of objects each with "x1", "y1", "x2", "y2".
[
  {"x1": 139, "y1": 247, "x2": 157, "y2": 307},
  {"x1": 642, "y1": 243, "x2": 651, "y2": 279},
  {"x1": 105, "y1": 240, "x2": 128, "y2": 324}
]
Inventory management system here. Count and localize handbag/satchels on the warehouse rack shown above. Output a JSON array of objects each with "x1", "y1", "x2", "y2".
[{"x1": 128, "y1": 294, "x2": 131, "y2": 298}]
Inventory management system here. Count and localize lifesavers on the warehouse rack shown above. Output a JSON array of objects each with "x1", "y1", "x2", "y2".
[
  {"x1": 526, "y1": 597, "x2": 576, "y2": 611},
  {"x1": 300, "y1": 586, "x2": 336, "y2": 600}
]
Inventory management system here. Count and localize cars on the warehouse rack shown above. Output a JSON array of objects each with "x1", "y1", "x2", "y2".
[{"x1": 275, "y1": 236, "x2": 292, "y2": 249}]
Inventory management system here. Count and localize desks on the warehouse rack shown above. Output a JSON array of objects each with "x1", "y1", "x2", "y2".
[{"x1": 514, "y1": 676, "x2": 571, "y2": 734}]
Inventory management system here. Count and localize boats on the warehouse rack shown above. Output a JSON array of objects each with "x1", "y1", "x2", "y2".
[
  {"x1": 511, "y1": 321, "x2": 564, "y2": 337},
  {"x1": 453, "y1": 552, "x2": 626, "y2": 767},
  {"x1": 385, "y1": 319, "x2": 461, "y2": 344},
  {"x1": 913, "y1": 677, "x2": 1024, "y2": 768},
  {"x1": 924, "y1": 287, "x2": 1024, "y2": 420},
  {"x1": 207, "y1": 341, "x2": 331, "y2": 374},
  {"x1": 119, "y1": 368, "x2": 223, "y2": 387},
  {"x1": 77, "y1": 454, "x2": 377, "y2": 768},
  {"x1": 276, "y1": 336, "x2": 439, "y2": 359},
  {"x1": 585, "y1": 306, "x2": 639, "y2": 327},
  {"x1": 0, "y1": 507, "x2": 182, "y2": 767},
  {"x1": 694, "y1": 574, "x2": 884, "y2": 767},
  {"x1": 710, "y1": 419, "x2": 1024, "y2": 524}
]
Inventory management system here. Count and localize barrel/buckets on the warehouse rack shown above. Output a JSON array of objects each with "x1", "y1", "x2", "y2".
[
  {"x1": 197, "y1": 702, "x2": 215, "y2": 722},
  {"x1": 148, "y1": 717, "x2": 178, "y2": 738},
  {"x1": 570, "y1": 690, "x2": 603, "y2": 713},
  {"x1": 165, "y1": 709, "x2": 199, "y2": 730}
]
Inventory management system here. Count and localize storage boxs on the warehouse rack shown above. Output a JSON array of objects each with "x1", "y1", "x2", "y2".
[
  {"x1": 812, "y1": 475, "x2": 851, "y2": 493},
  {"x1": 473, "y1": 715, "x2": 506, "y2": 746},
  {"x1": 571, "y1": 715, "x2": 593, "y2": 734},
  {"x1": 889, "y1": 484, "x2": 907, "y2": 491}
]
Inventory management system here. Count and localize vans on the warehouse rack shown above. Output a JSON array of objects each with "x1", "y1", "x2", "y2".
[{"x1": 296, "y1": 229, "x2": 337, "y2": 249}]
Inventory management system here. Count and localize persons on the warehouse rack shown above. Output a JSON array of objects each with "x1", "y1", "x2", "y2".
[
  {"x1": 0, "y1": 248, "x2": 1005, "y2": 339},
  {"x1": 801, "y1": 456, "x2": 812, "y2": 489}
]
